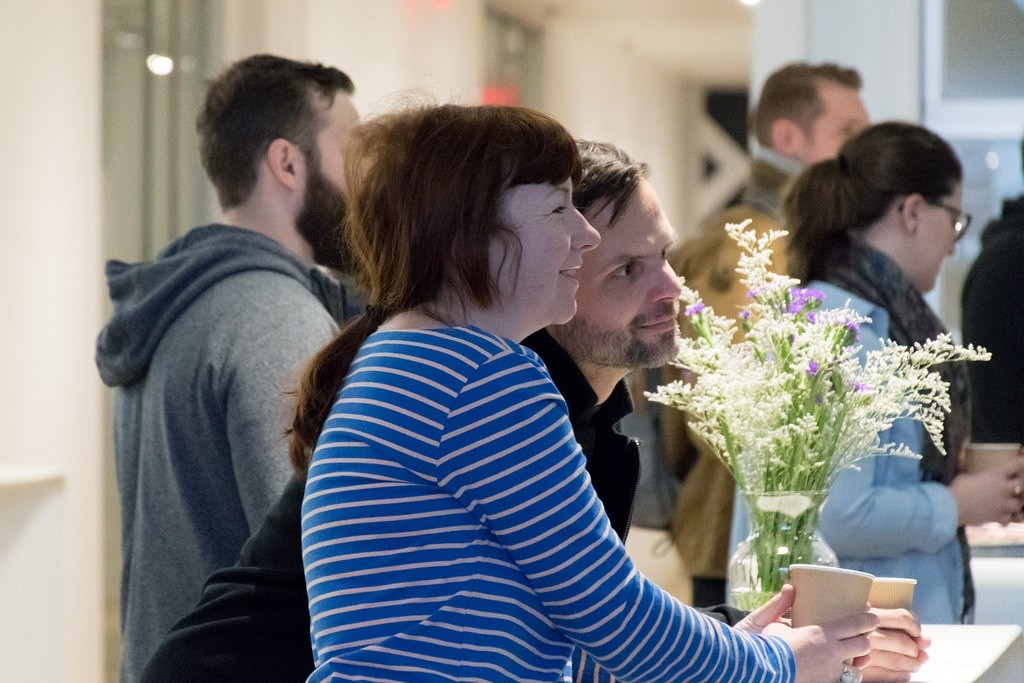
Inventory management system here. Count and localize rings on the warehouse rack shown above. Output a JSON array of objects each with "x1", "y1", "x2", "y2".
[
  {"x1": 842, "y1": 663, "x2": 858, "y2": 683},
  {"x1": 1006, "y1": 476, "x2": 1023, "y2": 499}
]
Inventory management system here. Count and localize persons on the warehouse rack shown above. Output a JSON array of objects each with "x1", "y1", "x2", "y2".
[
  {"x1": 720, "y1": 120, "x2": 1024, "y2": 624},
  {"x1": 95, "y1": 54, "x2": 366, "y2": 678},
  {"x1": 957, "y1": 118, "x2": 1023, "y2": 447},
  {"x1": 283, "y1": 101, "x2": 882, "y2": 683},
  {"x1": 135, "y1": 52, "x2": 931, "y2": 683},
  {"x1": 618, "y1": 404, "x2": 684, "y2": 532},
  {"x1": 643, "y1": 60, "x2": 877, "y2": 608}
]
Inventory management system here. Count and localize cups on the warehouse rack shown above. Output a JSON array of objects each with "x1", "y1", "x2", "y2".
[
  {"x1": 786, "y1": 563, "x2": 875, "y2": 668},
  {"x1": 864, "y1": 577, "x2": 917, "y2": 611},
  {"x1": 965, "y1": 442, "x2": 1020, "y2": 475}
]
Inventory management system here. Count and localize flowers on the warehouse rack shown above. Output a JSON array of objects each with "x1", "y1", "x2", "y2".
[{"x1": 640, "y1": 220, "x2": 996, "y2": 603}]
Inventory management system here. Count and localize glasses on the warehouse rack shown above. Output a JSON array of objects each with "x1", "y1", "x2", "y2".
[{"x1": 901, "y1": 195, "x2": 972, "y2": 244}]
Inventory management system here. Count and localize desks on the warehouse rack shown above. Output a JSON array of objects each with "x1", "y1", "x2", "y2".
[{"x1": 895, "y1": 622, "x2": 1024, "y2": 683}]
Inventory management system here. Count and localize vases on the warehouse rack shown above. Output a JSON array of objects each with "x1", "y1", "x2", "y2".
[{"x1": 719, "y1": 488, "x2": 840, "y2": 635}]
